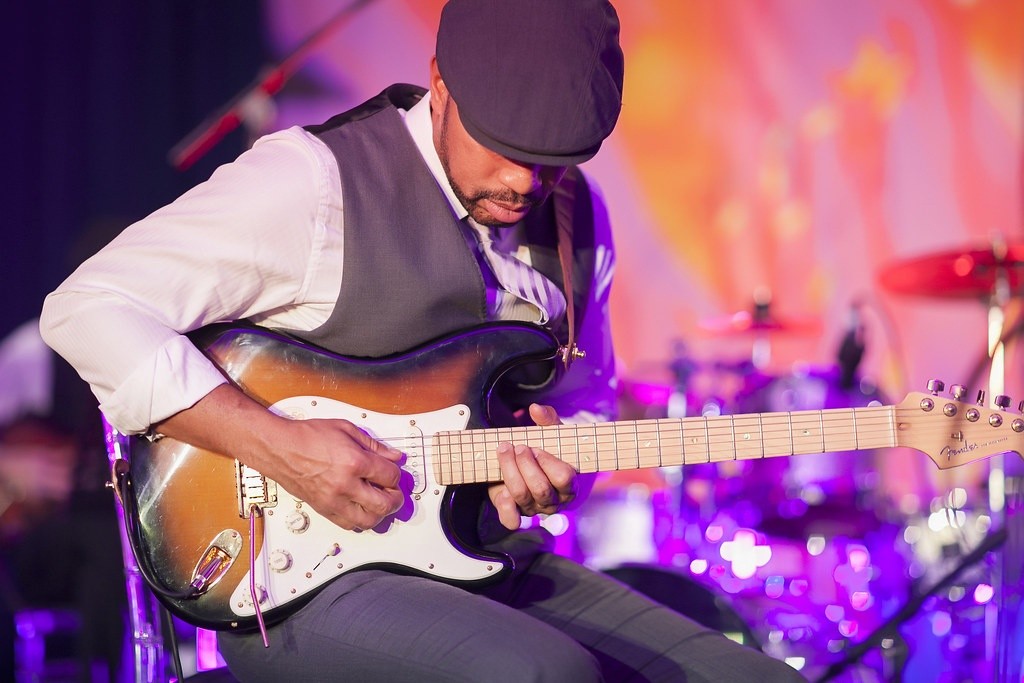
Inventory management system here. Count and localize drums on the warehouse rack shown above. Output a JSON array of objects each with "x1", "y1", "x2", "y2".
[
  {"x1": 617, "y1": 359, "x2": 763, "y2": 417},
  {"x1": 728, "y1": 364, "x2": 896, "y2": 539}
]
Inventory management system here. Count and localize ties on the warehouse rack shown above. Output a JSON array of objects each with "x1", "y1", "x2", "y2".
[{"x1": 466, "y1": 217, "x2": 566, "y2": 335}]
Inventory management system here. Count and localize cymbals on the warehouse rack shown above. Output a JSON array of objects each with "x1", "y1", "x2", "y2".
[{"x1": 876, "y1": 247, "x2": 1024, "y2": 304}]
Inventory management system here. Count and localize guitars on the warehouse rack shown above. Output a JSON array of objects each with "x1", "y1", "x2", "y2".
[{"x1": 114, "y1": 316, "x2": 1023, "y2": 638}]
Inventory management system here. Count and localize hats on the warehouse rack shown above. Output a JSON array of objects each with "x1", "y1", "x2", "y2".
[{"x1": 436, "y1": 0, "x2": 624, "y2": 168}]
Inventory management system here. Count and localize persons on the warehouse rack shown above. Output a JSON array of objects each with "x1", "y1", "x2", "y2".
[{"x1": 39, "y1": 0, "x2": 805, "y2": 683}]
